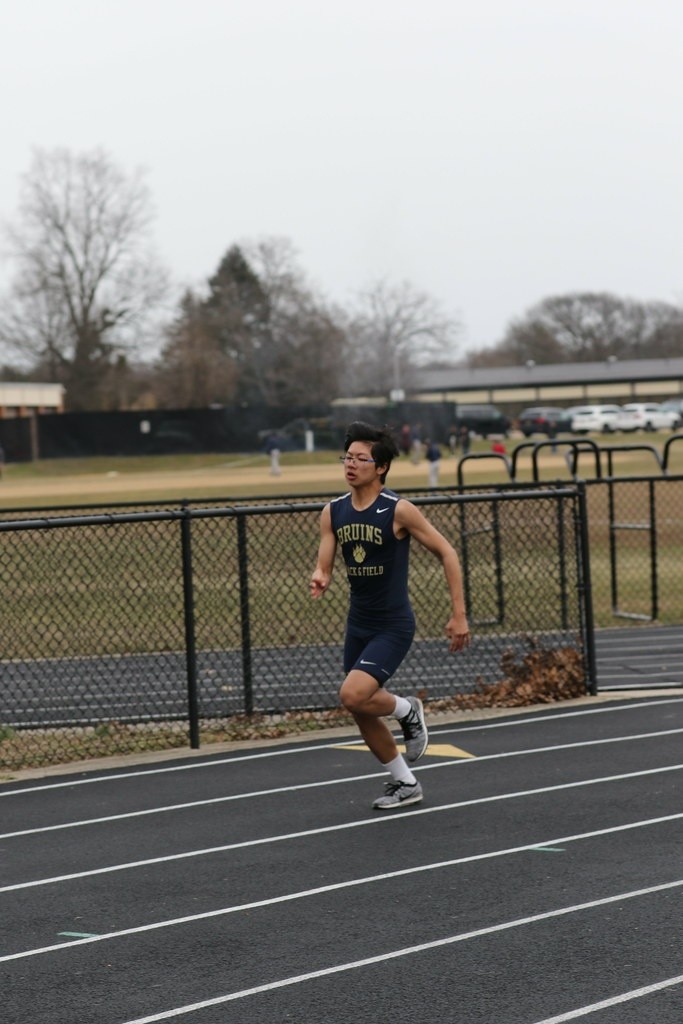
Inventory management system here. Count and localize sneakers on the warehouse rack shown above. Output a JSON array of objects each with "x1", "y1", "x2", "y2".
[
  {"x1": 372, "y1": 779, "x2": 423, "y2": 809},
  {"x1": 398, "y1": 696, "x2": 428, "y2": 762}
]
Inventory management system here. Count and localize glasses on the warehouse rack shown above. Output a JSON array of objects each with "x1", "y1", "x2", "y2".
[{"x1": 339, "y1": 456, "x2": 376, "y2": 465}]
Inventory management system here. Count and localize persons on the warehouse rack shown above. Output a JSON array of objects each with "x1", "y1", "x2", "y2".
[
  {"x1": 399, "y1": 424, "x2": 469, "y2": 488},
  {"x1": 265, "y1": 430, "x2": 284, "y2": 474},
  {"x1": 308, "y1": 422, "x2": 470, "y2": 809}
]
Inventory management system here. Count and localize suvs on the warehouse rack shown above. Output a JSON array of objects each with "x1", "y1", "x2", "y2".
[
  {"x1": 256, "y1": 416, "x2": 346, "y2": 455},
  {"x1": 455, "y1": 405, "x2": 510, "y2": 437}
]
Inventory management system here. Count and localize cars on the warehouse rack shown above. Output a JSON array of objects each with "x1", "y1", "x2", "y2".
[{"x1": 516, "y1": 400, "x2": 683, "y2": 439}]
